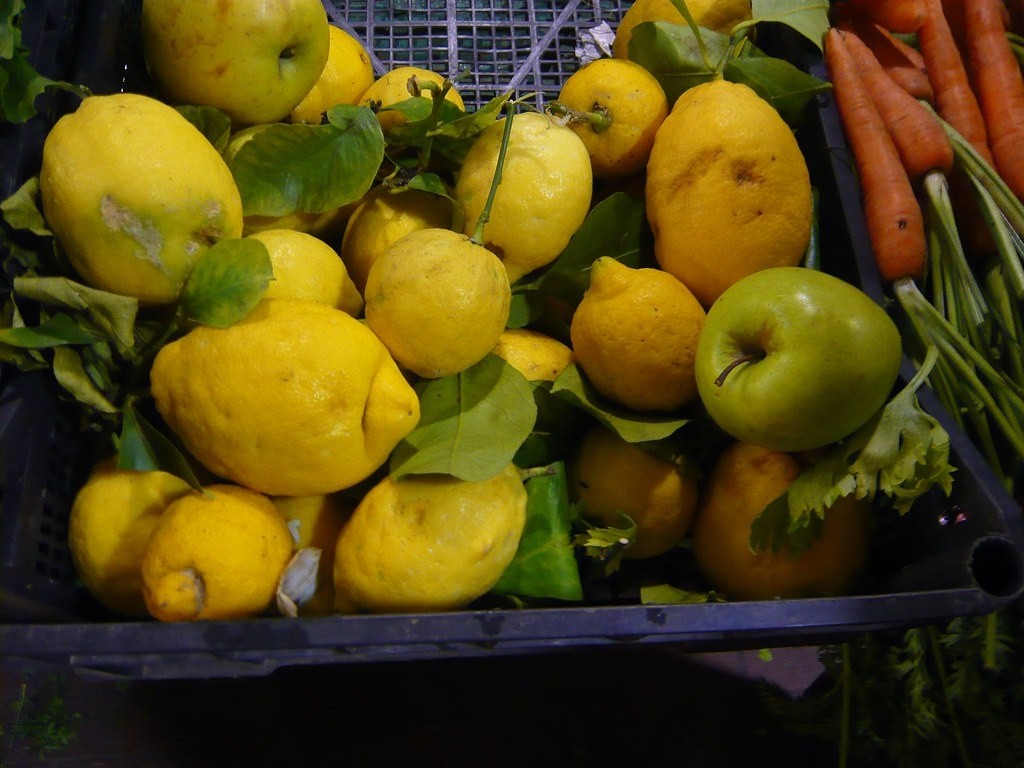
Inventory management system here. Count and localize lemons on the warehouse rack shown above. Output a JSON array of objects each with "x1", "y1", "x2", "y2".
[{"x1": 42, "y1": 0, "x2": 858, "y2": 619}]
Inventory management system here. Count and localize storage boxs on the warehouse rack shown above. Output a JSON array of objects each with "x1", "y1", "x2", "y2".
[{"x1": 1, "y1": 1, "x2": 1024, "y2": 690}]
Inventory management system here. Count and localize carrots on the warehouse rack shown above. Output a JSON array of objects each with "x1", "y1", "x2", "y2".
[{"x1": 824, "y1": 0, "x2": 1024, "y2": 278}]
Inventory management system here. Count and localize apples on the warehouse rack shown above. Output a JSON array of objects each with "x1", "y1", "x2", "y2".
[
  {"x1": 146, "y1": 1, "x2": 332, "y2": 124},
  {"x1": 696, "y1": 268, "x2": 901, "y2": 448}
]
